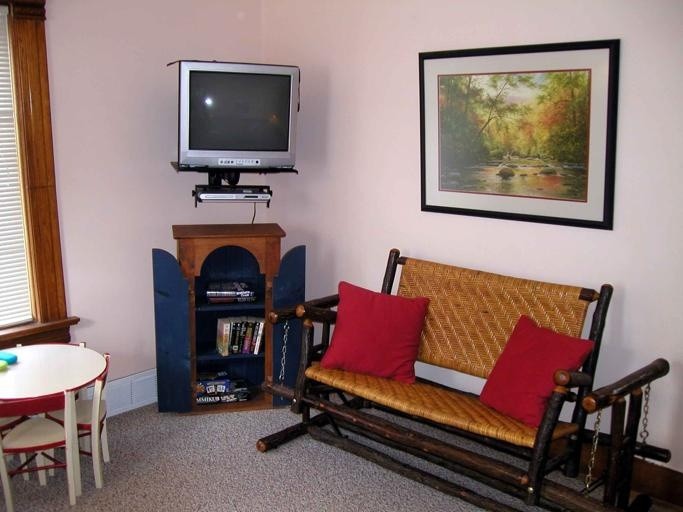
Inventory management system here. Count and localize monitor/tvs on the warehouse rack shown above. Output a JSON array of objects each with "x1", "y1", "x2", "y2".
[{"x1": 179, "y1": 60, "x2": 298, "y2": 169}]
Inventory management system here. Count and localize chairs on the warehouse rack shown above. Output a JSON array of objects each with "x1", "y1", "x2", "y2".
[{"x1": 0, "y1": 342, "x2": 112, "y2": 512}]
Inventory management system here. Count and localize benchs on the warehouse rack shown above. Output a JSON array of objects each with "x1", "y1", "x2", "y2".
[{"x1": 256, "y1": 249, "x2": 671, "y2": 512}]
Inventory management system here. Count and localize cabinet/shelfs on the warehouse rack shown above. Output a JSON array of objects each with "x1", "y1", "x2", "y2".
[{"x1": 151, "y1": 223, "x2": 305, "y2": 414}]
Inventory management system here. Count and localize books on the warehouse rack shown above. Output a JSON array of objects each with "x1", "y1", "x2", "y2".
[
  {"x1": 216, "y1": 315, "x2": 266, "y2": 357},
  {"x1": 206, "y1": 280, "x2": 257, "y2": 305}
]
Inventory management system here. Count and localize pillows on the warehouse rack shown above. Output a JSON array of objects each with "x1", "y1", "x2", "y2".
[
  {"x1": 321, "y1": 281, "x2": 430, "y2": 383},
  {"x1": 480, "y1": 315, "x2": 595, "y2": 428}
]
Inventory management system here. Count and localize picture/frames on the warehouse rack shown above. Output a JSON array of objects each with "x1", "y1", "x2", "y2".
[{"x1": 417, "y1": 38, "x2": 621, "y2": 231}]
